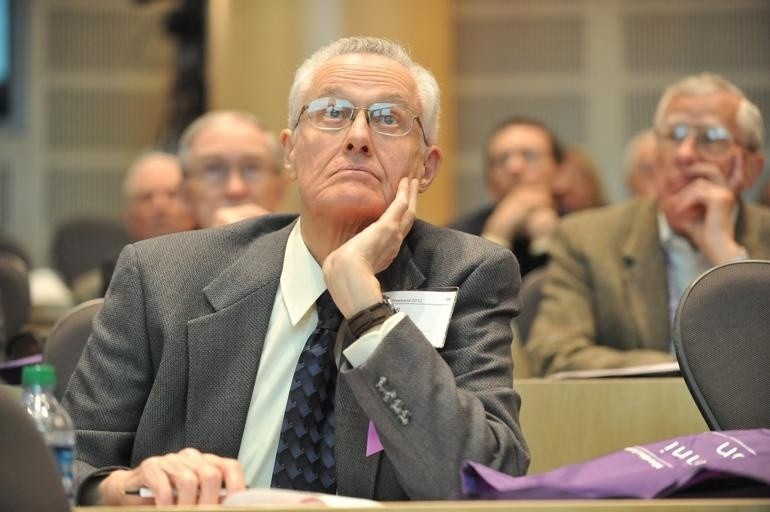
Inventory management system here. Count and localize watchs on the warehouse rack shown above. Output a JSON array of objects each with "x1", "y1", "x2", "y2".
[{"x1": 346, "y1": 295, "x2": 398, "y2": 338}]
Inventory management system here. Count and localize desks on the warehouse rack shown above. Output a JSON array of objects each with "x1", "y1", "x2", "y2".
[{"x1": 508, "y1": 368, "x2": 710, "y2": 479}]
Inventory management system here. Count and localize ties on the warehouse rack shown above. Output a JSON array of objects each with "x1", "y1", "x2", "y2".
[{"x1": 272, "y1": 291, "x2": 344, "y2": 492}]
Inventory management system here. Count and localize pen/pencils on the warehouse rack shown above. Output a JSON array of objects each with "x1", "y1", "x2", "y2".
[{"x1": 125, "y1": 486, "x2": 250, "y2": 499}]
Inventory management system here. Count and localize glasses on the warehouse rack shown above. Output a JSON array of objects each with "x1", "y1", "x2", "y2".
[
  {"x1": 293, "y1": 97, "x2": 430, "y2": 148},
  {"x1": 652, "y1": 120, "x2": 757, "y2": 154}
]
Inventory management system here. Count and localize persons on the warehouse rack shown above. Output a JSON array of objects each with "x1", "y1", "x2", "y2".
[
  {"x1": 121, "y1": 150, "x2": 196, "y2": 239},
  {"x1": 623, "y1": 127, "x2": 656, "y2": 194},
  {"x1": 520, "y1": 73, "x2": 770, "y2": 378},
  {"x1": 55, "y1": 35, "x2": 530, "y2": 507},
  {"x1": 98, "y1": 107, "x2": 279, "y2": 298},
  {"x1": 439, "y1": 117, "x2": 609, "y2": 279}
]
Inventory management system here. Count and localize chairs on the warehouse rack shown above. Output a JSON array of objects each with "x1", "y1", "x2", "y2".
[
  {"x1": 40, "y1": 294, "x2": 105, "y2": 407},
  {"x1": 671, "y1": 257, "x2": 770, "y2": 432},
  {"x1": 2, "y1": 387, "x2": 74, "y2": 511},
  {"x1": 517, "y1": 269, "x2": 550, "y2": 344}
]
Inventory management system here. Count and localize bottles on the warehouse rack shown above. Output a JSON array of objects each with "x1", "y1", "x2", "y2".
[{"x1": 16, "y1": 363, "x2": 75, "y2": 507}]
